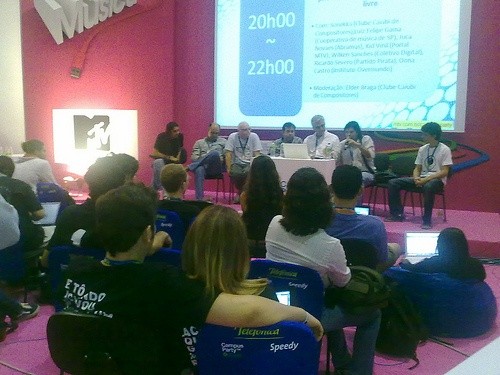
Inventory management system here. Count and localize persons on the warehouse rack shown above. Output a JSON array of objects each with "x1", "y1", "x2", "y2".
[
  {"x1": 185, "y1": 121, "x2": 227, "y2": 199},
  {"x1": 223, "y1": 121, "x2": 264, "y2": 204},
  {"x1": 150, "y1": 122, "x2": 187, "y2": 191},
  {"x1": 335, "y1": 121, "x2": 377, "y2": 208},
  {"x1": 0, "y1": 140, "x2": 485, "y2": 375},
  {"x1": 304, "y1": 115, "x2": 340, "y2": 159},
  {"x1": 274, "y1": 122, "x2": 302, "y2": 157},
  {"x1": 384, "y1": 122, "x2": 453, "y2": 229}
]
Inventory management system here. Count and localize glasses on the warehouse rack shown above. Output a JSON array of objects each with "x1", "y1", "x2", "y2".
[{"x1": 240, "y1": 127, "x2": 251, "y2": 131}]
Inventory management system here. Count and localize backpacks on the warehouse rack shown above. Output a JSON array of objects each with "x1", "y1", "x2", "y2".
[{"x1": 374, "y1": 283, "x2": 429, "y2": 358}]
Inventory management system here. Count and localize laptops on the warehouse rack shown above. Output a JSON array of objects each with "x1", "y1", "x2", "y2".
[
  {"x1": 274, "y1": 286, "x2": 298, "y2": 307},
  {"x1": 282, "y1": 143, "x2": 310, "y2": 159},
  {"x1": 354, "y1": 205, "x2": 370, "y2": 216},
  {"x1": 403, "y1": 232, "x2": 440, "y2": 263}
]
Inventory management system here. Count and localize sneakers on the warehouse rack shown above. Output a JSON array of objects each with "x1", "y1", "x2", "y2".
[{"x1": 11, "y1": 302, "x2": 40, "y2": 323}]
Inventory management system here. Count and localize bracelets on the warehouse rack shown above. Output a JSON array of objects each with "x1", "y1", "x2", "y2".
[{"x1": 304, "y1": 312, "x2": 309, "y2": 324}]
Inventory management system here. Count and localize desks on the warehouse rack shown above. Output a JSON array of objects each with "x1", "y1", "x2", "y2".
[{"x1": 250, "y1": 158, "x2": 336, "y2": 194}]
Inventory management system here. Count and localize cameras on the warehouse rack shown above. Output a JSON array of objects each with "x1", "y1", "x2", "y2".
[{"x1": 344, "y1": 142, "x2": 349, "y2": 150}]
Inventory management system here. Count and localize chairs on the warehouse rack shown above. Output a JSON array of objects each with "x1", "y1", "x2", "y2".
[
  {"x1": 0, "y1": 246, "x2": 25, "y2": 289},
  {"x1": 157, "y1": 248, "x2": 184, "y2": 268},
  {"x1": 37, "y1": 181, "x2": 75, "y2": 216},
  {"x1": 156, "y1": 209, "x2": 185, "y2": 252},
  {"x1": 367, "y1": 153, "x2": 449, "y2": 221},
  {"x1": 341, "y1": 239, "x2": 378, "y2": 269},
  {"x1": 249, "y1": 257, "x2": 323, "y2": 320},
  {"x1": 180, "y1": 149, "x2": 241, "y2": 206},
  {"x1": 46, "y1": 247, "x2": 106, "y2": 314},
  {"x1": 46, "y1": 313, "x2": 116, "y2": 375},
  {"x1": 196, "y1": 323, "x2": 321, "y2": 375}
]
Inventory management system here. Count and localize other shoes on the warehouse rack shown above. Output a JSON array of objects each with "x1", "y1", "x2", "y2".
[
  {"x1": 233, "y1": 198, "x2": 240, "y2": 204},
  {"x1": 383, "y1": 213, "x2": 403, "y2": 221},
  {"x1": 422, "y1": 220, "x2": 432, "y2": 228}
]
